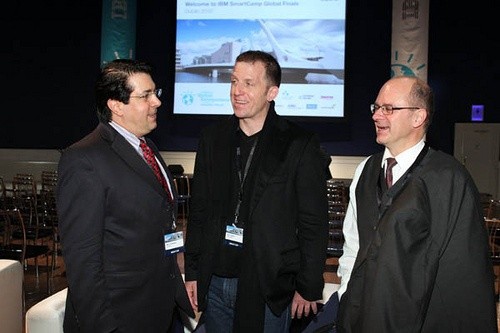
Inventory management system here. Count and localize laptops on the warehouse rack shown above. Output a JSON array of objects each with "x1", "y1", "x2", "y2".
[{"x1": 471, "y1": 104, "x2": 486, "y2": 122}]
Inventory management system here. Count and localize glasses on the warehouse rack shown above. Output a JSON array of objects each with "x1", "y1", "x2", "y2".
[
  {"x1": 371, "y1": 104, "x2": 421, "y2": 115},
  {"x1": 116, "y1": 88, "x2": 162, "y2": 102}
]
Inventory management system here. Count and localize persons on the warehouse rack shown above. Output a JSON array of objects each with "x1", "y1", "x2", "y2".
[
  {"x1": 43, "y1": 59, "x2": 192, "y2": 332},
  {"x1": 325, "y1": 73, "x2": 496, "y2": 333},
  {"x1": 185, "y1": 51, "x2": 326, "y2": 333}
]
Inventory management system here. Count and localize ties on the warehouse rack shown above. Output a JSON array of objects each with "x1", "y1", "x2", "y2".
[
  {"x1": 140, "y1": 139, "x2": 173, "y2": 206},
  {"x1": 386, "y1": 158, "x2": 397, "y2": 189}
]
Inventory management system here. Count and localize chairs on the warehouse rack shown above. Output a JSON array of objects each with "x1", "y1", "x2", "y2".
[
  {"x1": 327, "y1": 180, "x2": 350, "y2": 257},
  {"x1": 173, "y1": 176, "x2": 192, "y2": 230},
  {"x1": 481, "y1": 199, "x2": 500, "y2": 259},
  {"x1": 0, "y1": 170, "x2": 60, "y2": 312}
]
